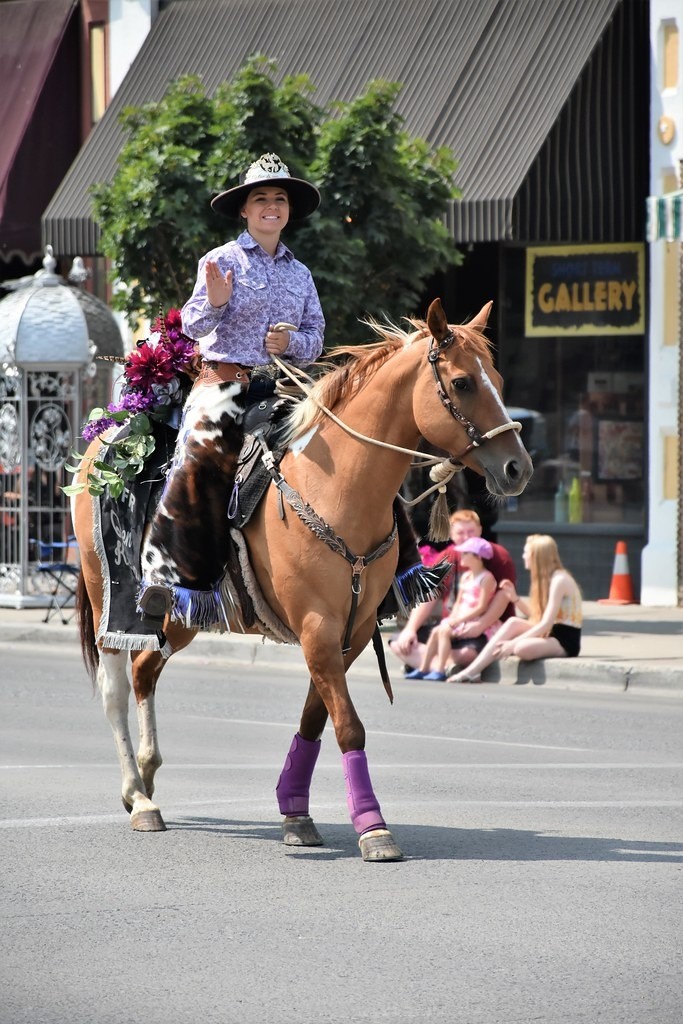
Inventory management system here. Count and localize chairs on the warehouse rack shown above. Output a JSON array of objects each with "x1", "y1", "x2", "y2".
[{"x1": 31, "y1": 534, "x2": 81, "y2": 624}]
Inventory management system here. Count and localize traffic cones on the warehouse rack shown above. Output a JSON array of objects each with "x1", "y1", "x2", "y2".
[{"x1": 594, "y1": 536, "x2": 639, "y2": 606}]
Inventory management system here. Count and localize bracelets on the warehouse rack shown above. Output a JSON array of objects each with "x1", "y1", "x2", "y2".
[{"x1": 512, "y1": 596, "x2": 520, "y2": 603}]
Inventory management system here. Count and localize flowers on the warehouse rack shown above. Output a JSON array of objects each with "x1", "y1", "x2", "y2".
[{"x1": 56, "y1": 303, "x2": 204, "y2": 498}]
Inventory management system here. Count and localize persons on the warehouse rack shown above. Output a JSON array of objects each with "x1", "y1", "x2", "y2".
[
  {"x1": 564, "y1": 395, "x2": 600, "y2": 505},
  {"x1": 135, "y1": 152, "x2": 325, "y2": 627},
  {"x1": 404, "y1": 535, "x2": 497, "y2": 680},
  {"x1": 4, "y1": 447, "x2": 61, "y2": 564},
  {"x1": 446, "y1": 534, "x2": 583, "y2": 683},
  {"x1": 388, "y1": 510, "x2": 516, "y2": 677}
]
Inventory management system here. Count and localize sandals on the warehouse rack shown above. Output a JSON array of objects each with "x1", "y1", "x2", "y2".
[{"x1": 446, "y1": 672, "x2": 481, "y2": 683}]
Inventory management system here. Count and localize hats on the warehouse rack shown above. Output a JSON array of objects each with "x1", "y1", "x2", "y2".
[
  {"x1": 454, "y1": 538, "x2": 494, "y2": 561},
  {"x1": 211, "y1": 151, "x2": 321, "y2": 222}
]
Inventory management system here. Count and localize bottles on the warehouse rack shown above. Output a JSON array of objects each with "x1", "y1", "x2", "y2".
[
  {"x1": 506, "y1": 496, "x2": 518, "y2": 512},
  {"x1": 569, "y1": 477, "x2": 583, "y2": 523},
  {"x1": 555, "y1": 480, "x2": 567, "y2": 522}
]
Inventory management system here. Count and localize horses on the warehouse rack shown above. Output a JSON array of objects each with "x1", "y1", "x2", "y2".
[{"x1": 66, "y1": 299, "x2": 534, "y2": 861}]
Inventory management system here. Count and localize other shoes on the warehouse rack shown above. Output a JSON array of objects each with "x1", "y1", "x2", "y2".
[
  {"x1": 404, "y1": 668, "x2": 428, "y2": 679},
  {"x1": 140, "y1": 585, "x2": 171, "y2": 630},
  {"x1": 422, "y1": 672, "x2": 446, "y2": 680}
]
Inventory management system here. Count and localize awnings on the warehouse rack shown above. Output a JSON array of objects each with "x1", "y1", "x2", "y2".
[{"x1": 0, "y1": 0, "x2": 650, "y2": 255}]
